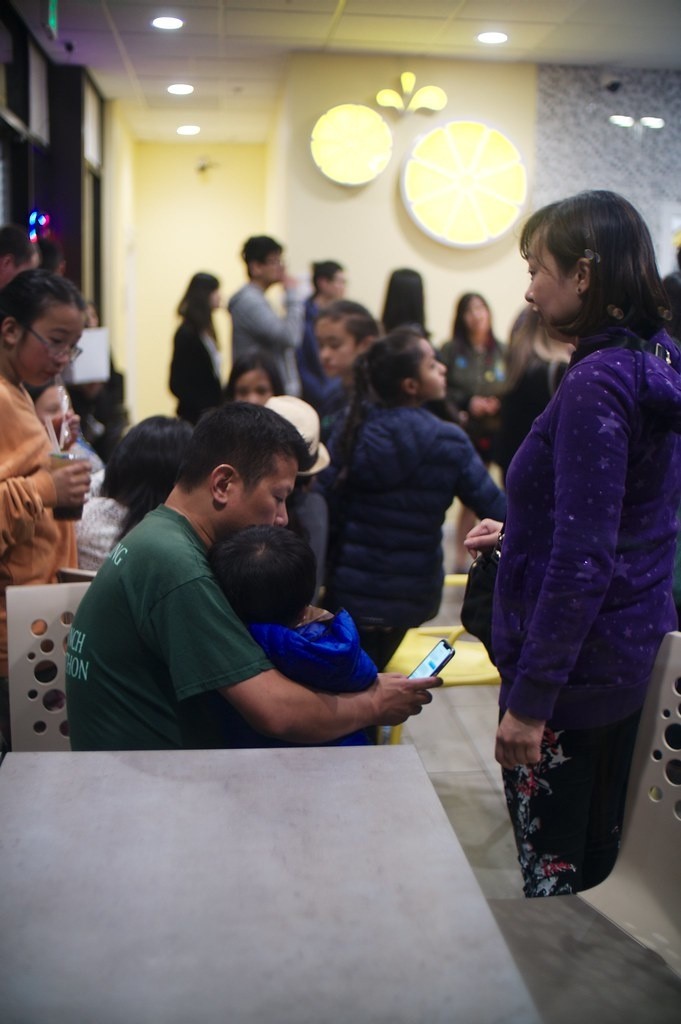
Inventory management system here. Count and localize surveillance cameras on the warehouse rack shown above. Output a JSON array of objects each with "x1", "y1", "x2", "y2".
[{"x1": 601, "y1": 74, "x2": 626, "y2": 91}]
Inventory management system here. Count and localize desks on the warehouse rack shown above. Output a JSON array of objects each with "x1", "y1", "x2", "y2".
[{"x1": 0, "y1": 740, "x2": 538, "y2": 1024}]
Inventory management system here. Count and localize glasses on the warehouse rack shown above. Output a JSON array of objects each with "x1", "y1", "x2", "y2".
[{"x1": 16, "y1": 319, "x2": 84, "y2": 364}]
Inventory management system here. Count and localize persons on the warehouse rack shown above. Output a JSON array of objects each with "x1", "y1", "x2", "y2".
[{"x1": 0, "y1": 191, "x2": 681, "y2": 898}]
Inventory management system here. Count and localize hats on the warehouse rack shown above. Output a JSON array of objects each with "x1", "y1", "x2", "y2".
[{"x1": 264, "y1": 395, "x2": 330, "y2": 477}]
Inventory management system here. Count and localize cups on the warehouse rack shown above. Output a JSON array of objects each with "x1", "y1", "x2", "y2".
[{"x1": 49, "y1": 452, "x2": 85, "y2": 520}]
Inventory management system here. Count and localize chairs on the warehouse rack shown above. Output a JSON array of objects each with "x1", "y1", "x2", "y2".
[
  {"x1": 488, "y1": 631, "x2": 681, "y2": 1024},
  {"x1": 6, "y1": 583, "x2": 100, "y2": 757}
]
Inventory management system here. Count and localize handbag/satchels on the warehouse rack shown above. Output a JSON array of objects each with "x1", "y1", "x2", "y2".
[{"x1": 461, "y1": 546, "x2": 497, "y2": 667}]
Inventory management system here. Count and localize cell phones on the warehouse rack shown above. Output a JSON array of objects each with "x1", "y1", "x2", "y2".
[{"x1": 407, "y1": 639, "x2": 456, "y2": 681}]
